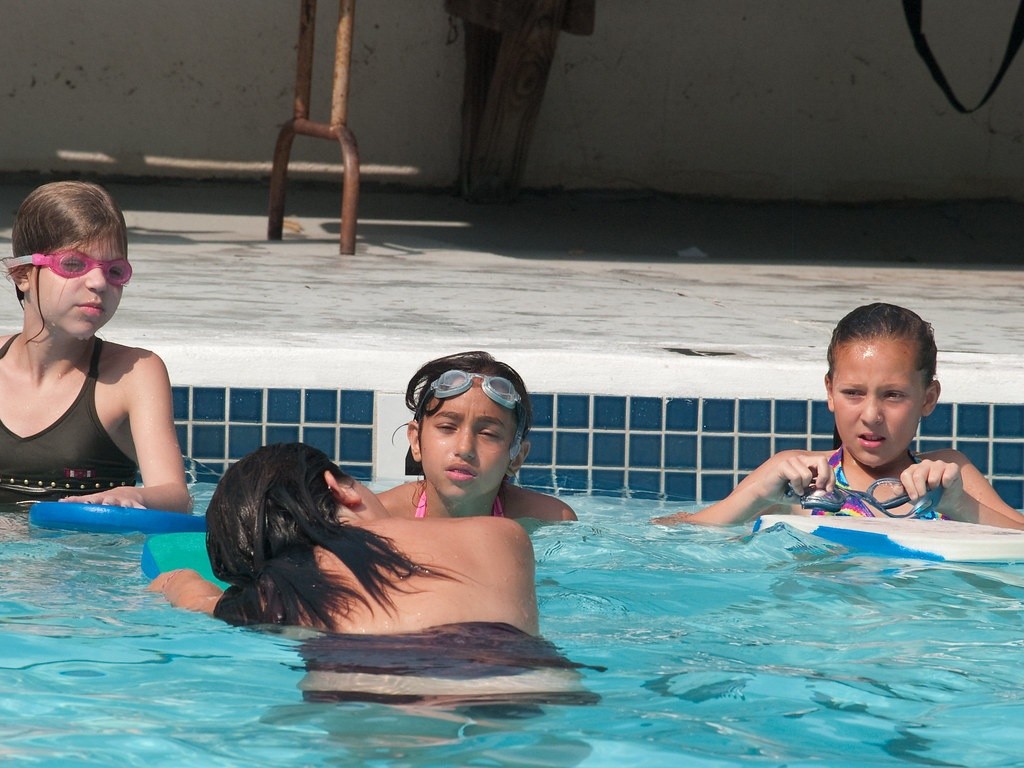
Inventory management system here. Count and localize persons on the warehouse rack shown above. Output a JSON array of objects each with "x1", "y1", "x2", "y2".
[
  {"x1": 0, "y1": 182, "x2": 196, "y2": 543},
  {"x1": 371, "y1": 351, "x2": 580, "y2": 522},
  {"x1": 148, "y1": 440, "x2": 608, "y2": 760},
  {"x1": 646, "y1": 302, "x2": 1024, "y2": 531}
]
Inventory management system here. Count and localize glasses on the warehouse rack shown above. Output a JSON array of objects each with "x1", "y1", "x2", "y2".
[
  {"x1": 800, "y1": 478, "x2": 911, "y2": 513},
  {"x1": 415, "y1": 369, "x2": 526, "y2": 460},
  {"x1": 1, "y1": 249, "x2": 132, "y2": 285}
]
